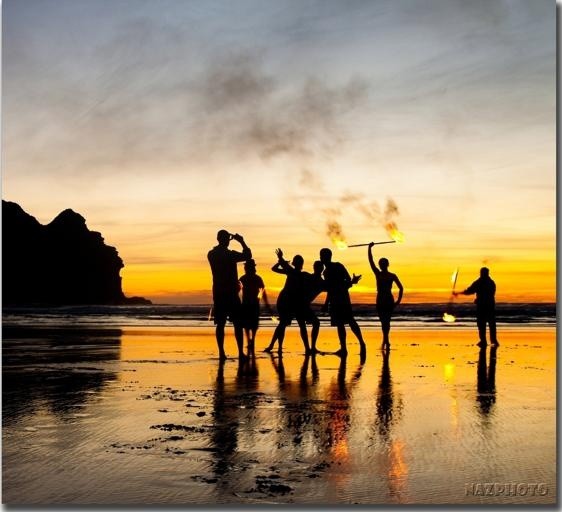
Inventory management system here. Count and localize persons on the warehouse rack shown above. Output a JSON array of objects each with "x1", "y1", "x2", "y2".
[
  {"x1": 453, "y1": 267, "x2": 500, "y2": 345},
  {"x1": 207, "y1": 229, "x2": 403, "y2": 362}
]
